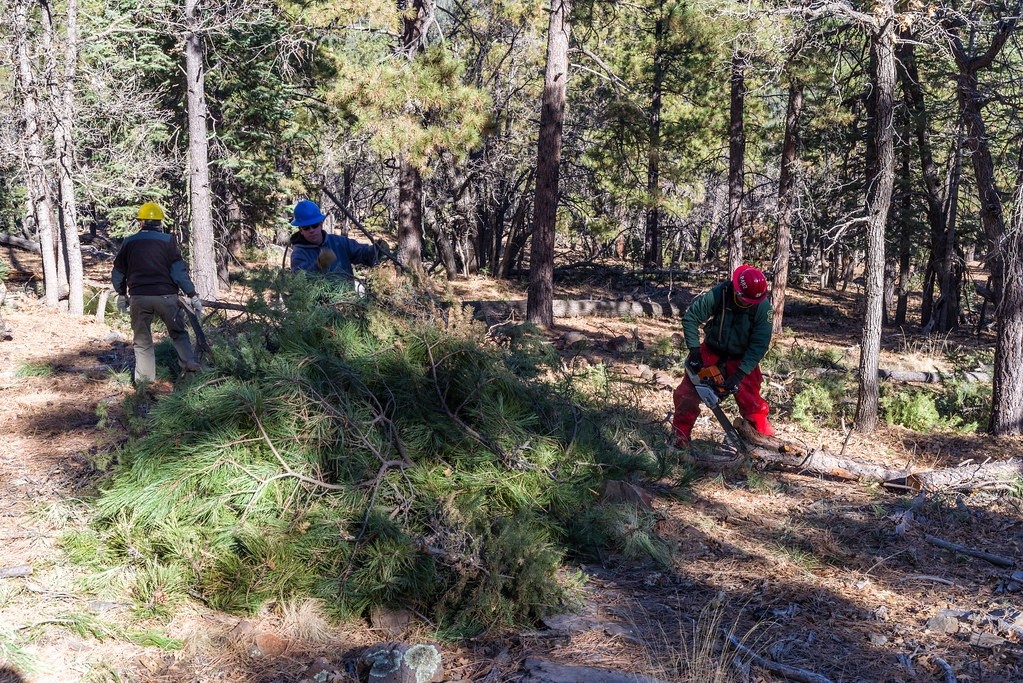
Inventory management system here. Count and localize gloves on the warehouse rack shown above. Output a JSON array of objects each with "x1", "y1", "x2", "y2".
[
  {"x1": 372, "y1": 238, "x2": 390, "y2": 258},
  {"x1": 723, "y1": 372, "x2": 742, "y2": 390},
  {"x1": 117, "y1": 295, "x2": 129, "y2": 309},
  {"x1": 315, "y1": 248, "x2": 337, "y2": 270},
  {"x1": 687, "y1": 349, "x2": 703, "y2": 374},
  {"x1": 191, "y1": 296, "x2": 202, "y2": 311}
]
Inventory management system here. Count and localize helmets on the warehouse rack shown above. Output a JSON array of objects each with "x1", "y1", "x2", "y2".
[
  {"x1": 135, "y1": 203, "x2": 164, "y2": 220},
  {"x1": 732, "y1": 265, "x2": 768, "y2": 304},
  {"x1": 291, "y1": 200, "x2": 325, "y2": 226}
]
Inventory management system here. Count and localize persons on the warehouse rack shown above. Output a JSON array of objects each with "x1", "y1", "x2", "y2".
[
  {"x1": 663, "y1": 264, "x2": 776, "y2": 468},
  {"x1": 108, "y1": 202, "x2": 202, "y2": 396},
  {"x1": 290, "y1": 200, "x2": 391, "y2": 342}
]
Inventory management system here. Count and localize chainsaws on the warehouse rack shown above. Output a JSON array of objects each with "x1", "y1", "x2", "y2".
[{"x1": 683, "y1": 343, "x2": 754, "y2": 459}]
